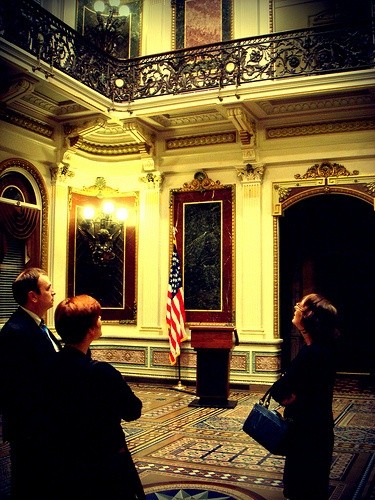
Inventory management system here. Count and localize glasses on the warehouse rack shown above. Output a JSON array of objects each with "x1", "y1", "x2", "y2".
[{"x1": 293, "y1": 302, "x2": 307, "y2": 315}]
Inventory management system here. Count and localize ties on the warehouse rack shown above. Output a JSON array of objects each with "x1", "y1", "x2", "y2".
[{"x1": 39, "y1": 322, "x2": 62, "y2": 353}]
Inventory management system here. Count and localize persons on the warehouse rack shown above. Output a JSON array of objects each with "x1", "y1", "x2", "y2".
[
  {"x1": 268, "y1": 294, "x2": 339, "y2": 500},
  {"x1": 0, "y1": 267, "x2": 62, "y2": 500},
  {"x1": 36, "y1": 295, "x2": 147, "y2": 500}
]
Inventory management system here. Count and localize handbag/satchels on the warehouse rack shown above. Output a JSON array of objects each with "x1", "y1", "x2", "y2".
[{"x1": 242, "y1": 378, "x2": 298, "y2": 455}]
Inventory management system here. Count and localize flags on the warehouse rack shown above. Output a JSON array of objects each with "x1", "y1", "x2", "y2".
[{"x1": 166, "y1": 238, "x2": 187, "y2": 366}]
